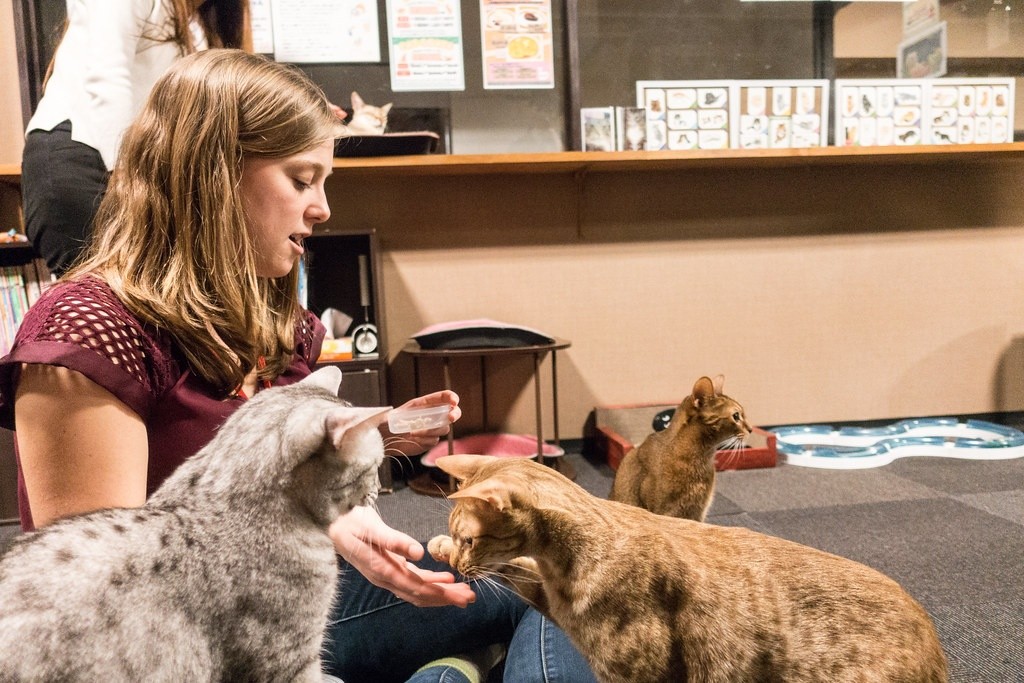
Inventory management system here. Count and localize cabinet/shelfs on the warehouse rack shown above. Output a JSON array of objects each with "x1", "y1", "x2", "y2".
[{"x1": 294, "y1": 229, "x2": 398, "y2": 497}]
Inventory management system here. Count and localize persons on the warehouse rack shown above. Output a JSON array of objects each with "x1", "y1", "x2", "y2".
[
  {"x1": 0, "y1": 48, "x2": 597, "y2": 683},
  {"x1": 22, "y1": 0, "x2": 226, "y2": 277}
]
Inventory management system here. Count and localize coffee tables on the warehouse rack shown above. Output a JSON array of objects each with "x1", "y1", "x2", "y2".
[{"x1": 402, "y1": 339, "x2": 573, "y2": 498}]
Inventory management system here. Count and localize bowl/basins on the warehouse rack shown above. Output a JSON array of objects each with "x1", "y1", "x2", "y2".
[{"x1": 388, "y1": 403, "x2": 452, "y2": 435}]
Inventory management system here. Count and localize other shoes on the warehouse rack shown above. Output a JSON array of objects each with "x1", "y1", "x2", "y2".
[{"x1": 401, "y1": 658, "x2": 481, "y2": 683}]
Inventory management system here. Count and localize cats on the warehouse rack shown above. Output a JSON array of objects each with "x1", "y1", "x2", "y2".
[
  {"x1": 331, "y1": 91, "x2": 394, "y2": 139},
  {"x1": 0, "y1": 365, "x2": 950, "y2": 683}
]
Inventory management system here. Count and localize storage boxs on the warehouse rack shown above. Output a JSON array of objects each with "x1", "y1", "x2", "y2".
[{"x1": 595, "y1": 403, "x2": 777, "y2": 477}]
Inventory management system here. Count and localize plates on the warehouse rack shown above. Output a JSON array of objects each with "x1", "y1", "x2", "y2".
[{"x1": 644, "y1": 85, "x2": 1008, "y2": 152}]
